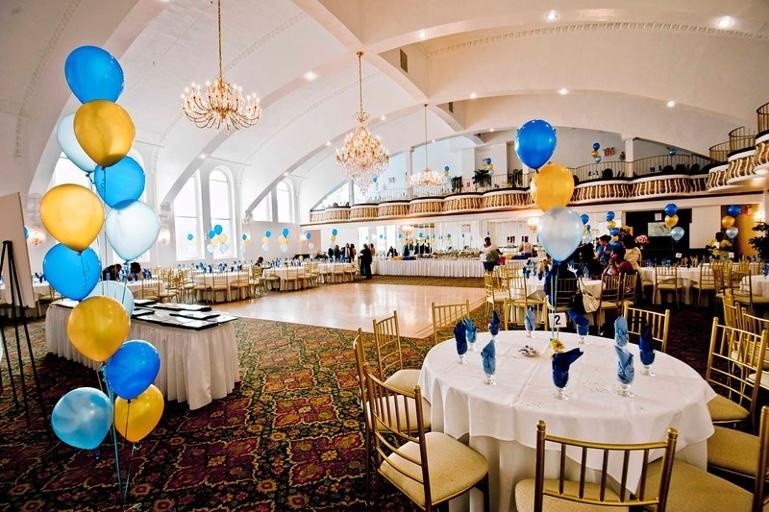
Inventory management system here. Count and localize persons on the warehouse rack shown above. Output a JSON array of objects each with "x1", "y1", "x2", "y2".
[
  {"x1": 254, "y1": 256, "x2": 264, "y2": 267},
  {"x1": 316, "y1": 240, "x2": 433, "y2": 281},
  {"x1": 706, "y1": 231, "x2": 735, "y2": 259},
  {"x1": 481, "y1": 234, "x2": 645, "y2": 327}
]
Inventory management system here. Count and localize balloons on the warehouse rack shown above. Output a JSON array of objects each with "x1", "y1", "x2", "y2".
[
  {"x1": 443, "y1": 166, "x2": 449, "y2": 178},
  {"x1": 664, "y1": 203, "x2": 684, "y2": 242},
  {"x1": 41, "y1": 183, "x2": 161, "y2": 316},
  {"x1": 51, "y1": 296, "x2": 165, "y2": 451},
  {"x1": 58, "y1": 45, "x2": 145, "y2": 208},
  {"x1": 188, "y1": 225, "x2": 465, "y2": 252},
  {"x1": 592, "y1": 142, "x2": 601, "y2": 164},
  {"x1": 486, "y1": 158, "x2": 495, "y2": 175},
  {"x1": 723, "y1": 206, "x2": 742, "y2": 240},
  {"x1": 516, "y1": 119, "x2": 590, "y2": 262}
]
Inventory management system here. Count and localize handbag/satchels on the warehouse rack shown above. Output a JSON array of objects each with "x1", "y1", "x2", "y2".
[{"x1": 573, "y1": 280, "x2": 606, "y2": 314}]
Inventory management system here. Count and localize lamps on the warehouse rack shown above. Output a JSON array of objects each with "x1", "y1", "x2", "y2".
[
  {"x1": 409, "y1": 103, "x2": 444, "y2": 187},
  {"x1": 179, "y1": 0, "x2": 261, "y2": 132},
  {"x1": 336, "y1": 52, "x2": 390, "y2": 194}
]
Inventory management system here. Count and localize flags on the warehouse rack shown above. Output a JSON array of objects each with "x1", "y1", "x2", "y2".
[{"x1": 605, "y1": 212, "x2": 620, "y2": 235}]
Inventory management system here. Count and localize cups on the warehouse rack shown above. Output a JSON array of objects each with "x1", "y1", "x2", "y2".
[
  {"x1": 618, "y1": 368, "x2": 633, "y2": 397},
  {"x1": 467, "y1": 333, "x2": 475, "y2": 351},
  {"x1": 489, "y1": 325, "x2": 499, "y2": 342},
  {"x1": 644, "y1": 252, "x2": 715, "y2": 274},
  {"x1": 617, "y1": 336, "x2": 628, "y2": 352},
  {"x1": 526, "y1": 320, "x2": 535, "y2": 338},
  {"x1": 578, "y1": 325, "x2": 589, "y2": 344},
  {"x1": 553, "y1": 371, "x2": 567, "y2": 400},
  {"x1": 640, "y1": 352, "x2": 655, "y2": 376},
  {"x1": 484, "y1": 360, "x2": 496, "y2": 386},
  {"x1": 457, "y1": 344, "x2": 468, "y2": 364}
]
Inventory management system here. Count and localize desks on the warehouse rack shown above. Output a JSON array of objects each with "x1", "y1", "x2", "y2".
[{"x1": 44, "y1": 297, "x2": 240, "y2": 412}]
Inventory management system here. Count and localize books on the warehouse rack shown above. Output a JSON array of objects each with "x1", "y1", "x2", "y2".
[{"x1": 131, "y1": 298, "x2": 240, "y2": 330}]
